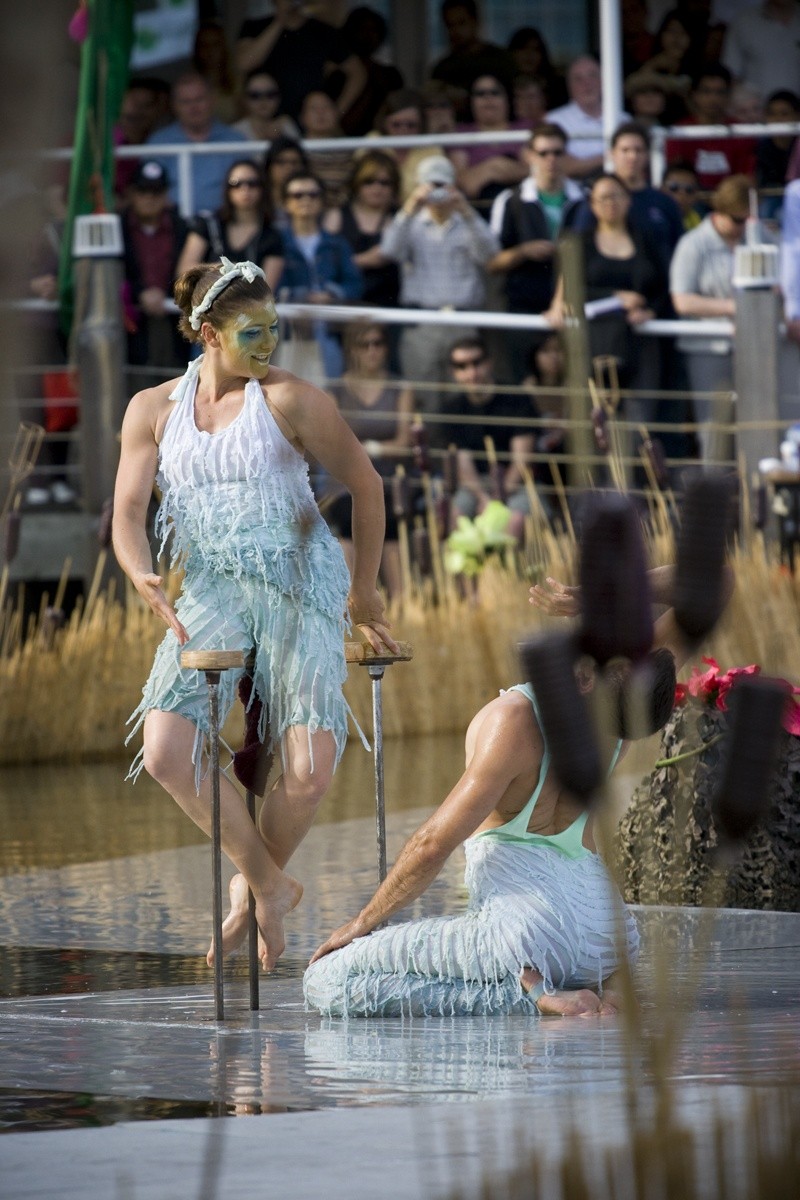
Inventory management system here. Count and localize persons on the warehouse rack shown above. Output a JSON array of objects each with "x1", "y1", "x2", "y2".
[
  {"x1": 10, "y1": 0, "x2": 800, "y2": 605},
  {"x1": 302, "y1": 564, "x2": 735, "y2": 1018},
  {"x1": 112, "y1": 256, "x2": 397, "y2": 970}
]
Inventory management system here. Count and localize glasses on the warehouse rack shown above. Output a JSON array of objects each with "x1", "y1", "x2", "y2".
[
  {"x1": 452, "y1": 355, "x2": 486, "y2": 371},
  {"x1": 355, "y1": 176, "x2": 394, "y2": 189},
  {"x1": 665, "y1": 179, "x2": 699, "y2": 195},
  {"x1": 269, "y1": 157, "x2": 307, "y2": 171},
  {"x1": 356, "y1": 338, "x2": 383, "y2": 349},
  {"x1": 474, "y1": 87, "x2": 501, "y2": 98},
  {"x1": 228, "y1": 176, "x2": 259, "y2": 191},
  {"x1": 288, "y1": 189, "x2": 322, "y2": 202},
  {"x1": 728, "y1": 213, "x2": 747, "y2": 225},
  {"x1": 246, "y1": 87, "x2": 278, "y2": 103},
  {"x1": 533, "y1": 145, "x2": 565, "y2": 159}
]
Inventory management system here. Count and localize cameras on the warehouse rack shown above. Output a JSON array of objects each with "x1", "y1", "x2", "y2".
[{"x1": 429, "y1": 180, "x2": 451, "y2": 199}]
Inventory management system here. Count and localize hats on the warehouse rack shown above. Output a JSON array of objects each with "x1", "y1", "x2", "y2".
[
  {"x1": 128, "y1": 160, "x2": 170, "y2": 196},
  {"x1": 416, "y1": 157, "x2": 456, "y2": 186}
]
